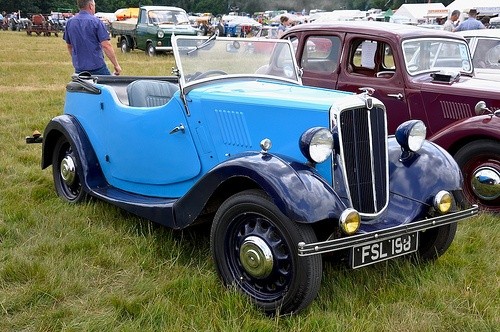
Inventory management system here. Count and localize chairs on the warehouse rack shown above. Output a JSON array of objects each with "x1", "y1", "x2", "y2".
[{"x1": 127, "y1": 79, "x2": 180, "y2": 107}]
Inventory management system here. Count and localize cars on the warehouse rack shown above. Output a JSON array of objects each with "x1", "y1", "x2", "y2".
[
  {"x1": 188, "y1": 9, "x2": 303, "y2": 50},
  {"x1": 391, "y1": 29, "x2": 500, "y2": 83},
  {"x1": 25, "y1": 35, "x2": 479, "y2": 321}
]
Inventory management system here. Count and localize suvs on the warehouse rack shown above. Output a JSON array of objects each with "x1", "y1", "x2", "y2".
[{"x1": 256, "y1": 21, "x2": 500, "y2": 214}]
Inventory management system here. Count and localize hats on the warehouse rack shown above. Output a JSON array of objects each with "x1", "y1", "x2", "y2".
[{"x1": 467, "y1": 9, "x2": 480, "y2": 15}]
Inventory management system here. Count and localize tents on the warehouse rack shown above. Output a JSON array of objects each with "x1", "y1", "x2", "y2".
[
  {"x1": 390, "y1": 3, "x2": 448, "y2": 24},
  {"x1": 270, "y1": 13, "x2": 304, "y2": 23},
  {"x1": 316, "y1": 10, "x2": 366, "y2": 21},
  {"x1": 446, "y1": 0, "x2": 500, "y2": 16},
  {"x1": 222, "y1": 15, "x2": 263, "y2": 38}
]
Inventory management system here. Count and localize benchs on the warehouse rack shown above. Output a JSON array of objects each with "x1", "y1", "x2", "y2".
[{"x1": 301, "y1": 60, "x2": 365, "y2": 78}]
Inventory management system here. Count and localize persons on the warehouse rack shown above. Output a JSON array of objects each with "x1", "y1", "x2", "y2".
[
  {"x1": 452, "y1": 8, "x2": 487, "y2": 32},
  {"x1": 277, "y1": 16, "x2": 289, "y2": 40},
  {"x1": 2, "y1": 11, "x2": 9, "y2": 25},
  {"x1": 65, "y1": 0, "x2": 122, "y2": 75},
  {"x1": 442, "y1": 10, "x2": 461, "y2": 57}
]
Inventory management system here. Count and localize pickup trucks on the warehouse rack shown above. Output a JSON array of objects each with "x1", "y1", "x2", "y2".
[{"x1": 112, "y1": 6, "x2": 203, "y2": 59}]
